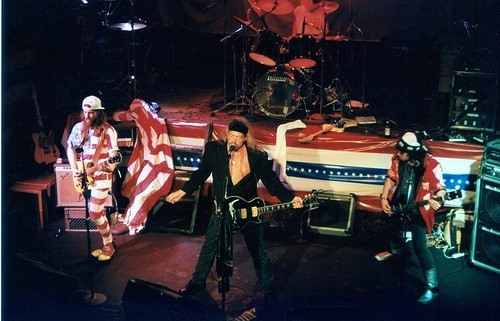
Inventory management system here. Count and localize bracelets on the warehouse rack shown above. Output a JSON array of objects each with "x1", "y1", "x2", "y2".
[{"x1": 380, "y1": 194, "x2": 388, "y2": 199}]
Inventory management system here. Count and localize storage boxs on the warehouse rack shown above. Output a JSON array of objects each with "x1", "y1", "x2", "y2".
[{"x1": 64, "y1": 208, "x2": 101, "y2": 233}]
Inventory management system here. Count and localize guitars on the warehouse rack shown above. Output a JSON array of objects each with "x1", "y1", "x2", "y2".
[
  {"x1": 298, "y1": 117, "x2": 359, "y2": 143},
  {"x1": 30, "y1": 87, "x2": 61, "y2": 165},
  {"x1": 73, "y1": 152, "x2": 123, "y2": 194},
  {"x1": 220, "y1": 191, "x2": 319, "y2": 233},
  {"x1": 378, "y1": 189, "x2": 463, "y2": 233}
]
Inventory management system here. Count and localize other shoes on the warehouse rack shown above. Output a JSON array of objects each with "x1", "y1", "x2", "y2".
[
  {"x1": 250, "y1": 278, "x2": 271, "y2": 293},
  {"x1": 179, "y1": 280, "x2": 206, "y2": 295},
  {"x1": 98, "y1": 249, "x2": 116, "y2": 260},
  {"x1": 91, "y1": 248, "x2": 104, "y2": 257},
  {"x1": 418, "y1": 289, "x2": 438, "y2": 302},
  {"x1": 375, "y1": 250, "x2": 394, "y2": 261}
]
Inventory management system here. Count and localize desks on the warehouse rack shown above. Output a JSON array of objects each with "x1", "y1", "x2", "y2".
[{"x1": 9, "y1": 171, "x2": 57, "y2": 229}]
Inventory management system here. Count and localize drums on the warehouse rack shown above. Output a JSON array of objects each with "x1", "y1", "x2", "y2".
[
  {"x1": 248, "y1": 29, "x2": 282, "y2": 67},
  {"x1": 288, "y1": 33, "x2": 316, "y2": 69},
  {"x1": 101, "y1": 0, "x2": 148, "y2": 31},
  {"x1": 254, "y1": 68, "x2": 310, "y2": 117}
]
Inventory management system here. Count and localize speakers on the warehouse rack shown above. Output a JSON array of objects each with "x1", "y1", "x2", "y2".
[
  {"x1": 54, "y1": 163, "x2": 115, "y2": 209},
  {"x1": 449, "y1": 70, "x2": 499, "y2": 132},
  {"x1": 306, "y1": 190, "x2": 357, "y2": 236},
  {"x1": 9, "y1": 250, "x2": 81, "y2": 305},
  {"x1": 149, "y1": 198, "x2": 205, "y2": 236},
  {"x1": 470, "y1": 175, "x2": 500, "y2": 274},
  {"x1": 122, "y1": 278, "x2": 219, "y2": 321}
]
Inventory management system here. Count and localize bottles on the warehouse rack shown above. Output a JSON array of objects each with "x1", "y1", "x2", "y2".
[{"x1": 385, "y1": 117, "x2": 391, "y2": 138}]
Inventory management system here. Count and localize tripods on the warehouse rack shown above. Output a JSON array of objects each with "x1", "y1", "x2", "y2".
[
  {"x1": 326, "y1": 41, "x2": 353, "y2": 113},
  {"x1": 211, "y1": 28, "x2": 260, "y2": 116}
]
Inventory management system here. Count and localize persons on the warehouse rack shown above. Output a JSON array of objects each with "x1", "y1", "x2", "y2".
[
  {"x1": 293, "y1": 0, "x2": 339, "y2": 37},
  {"x1": 166, "y1": 119, "x2": 304, "y2": 296},
  {"x1": 66, "y1": 96, "x2": 115, "y2": 260},
  {"x1": 369, "y1": 131, "x2": 446, "y2": 304}
]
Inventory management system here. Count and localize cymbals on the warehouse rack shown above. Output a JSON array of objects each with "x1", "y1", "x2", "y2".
[
  {"x1": 257, "y1": 0, "x2": 295, "y2": 16},
  {"x1": 309, "y1": 1, "x2": 339, "y2": 14}
]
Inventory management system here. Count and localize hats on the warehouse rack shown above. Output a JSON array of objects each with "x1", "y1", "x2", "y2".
[
  {"x1": 82, "y1": 95, "x2": 105, "y2": 110},
  {"x1": 398, "y1": 132, "x2": 422, "y2": 152}
]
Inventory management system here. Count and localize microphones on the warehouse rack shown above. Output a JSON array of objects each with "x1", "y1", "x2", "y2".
[
  {"x1": 347, "y1": 16, "x2": 358, "y2": 34},
  {"x1": 302, "y1": 17, "x2": 306, "y2": 34},
  {"x1": 227, "y1": 143, "x2": 236, "y2": 156},
  {"x1": 79, "y1": 136, "x2": 88, "y2": 149}
]
